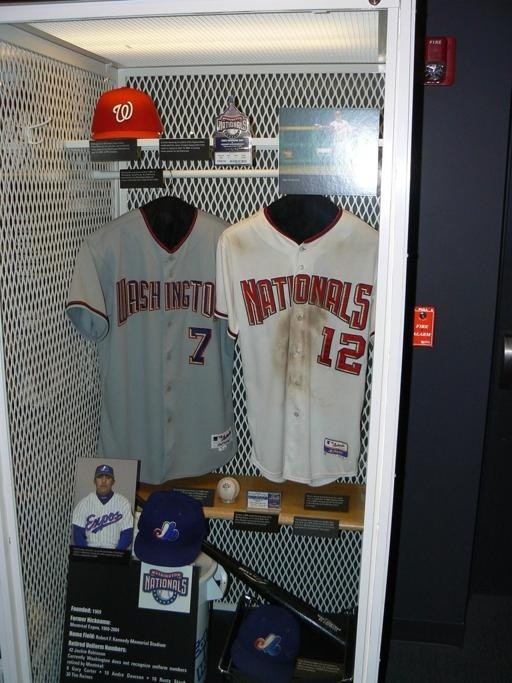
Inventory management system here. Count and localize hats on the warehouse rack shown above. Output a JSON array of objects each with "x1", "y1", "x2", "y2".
[
  {"x1": 94, "y1": 465, "x2": 115, "y2": 478},
  {"x1": 89, "y1": 86, "x2": 163, "y2": 141},
  {"x1": 133, "y1": 490, "x2": 209, "y2": 568},
  {"x1": 230, "y1": 605, "x2": 302, "y2": 683}
]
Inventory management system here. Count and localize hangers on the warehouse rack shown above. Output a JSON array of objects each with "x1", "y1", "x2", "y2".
[{"x1": 91, "y1": 169, "x2": 227, "y2": 239}]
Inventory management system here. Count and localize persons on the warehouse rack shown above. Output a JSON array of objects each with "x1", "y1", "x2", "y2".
[
  {"x1": 72, "y1": 464, "x2": 134, "y2": 550},
  {"x1": 314, "y1": 110, "x2": 351, "y2": 134}
]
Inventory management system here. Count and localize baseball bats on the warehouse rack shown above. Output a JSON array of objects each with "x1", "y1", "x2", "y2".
[{"x1": 135, "y1": 494, "x2": 347, "y2": 656}]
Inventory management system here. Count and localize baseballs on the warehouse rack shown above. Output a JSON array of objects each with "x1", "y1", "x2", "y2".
[{"x1": 216, "y1": 477, "x2": 240, "y2": 501}]
